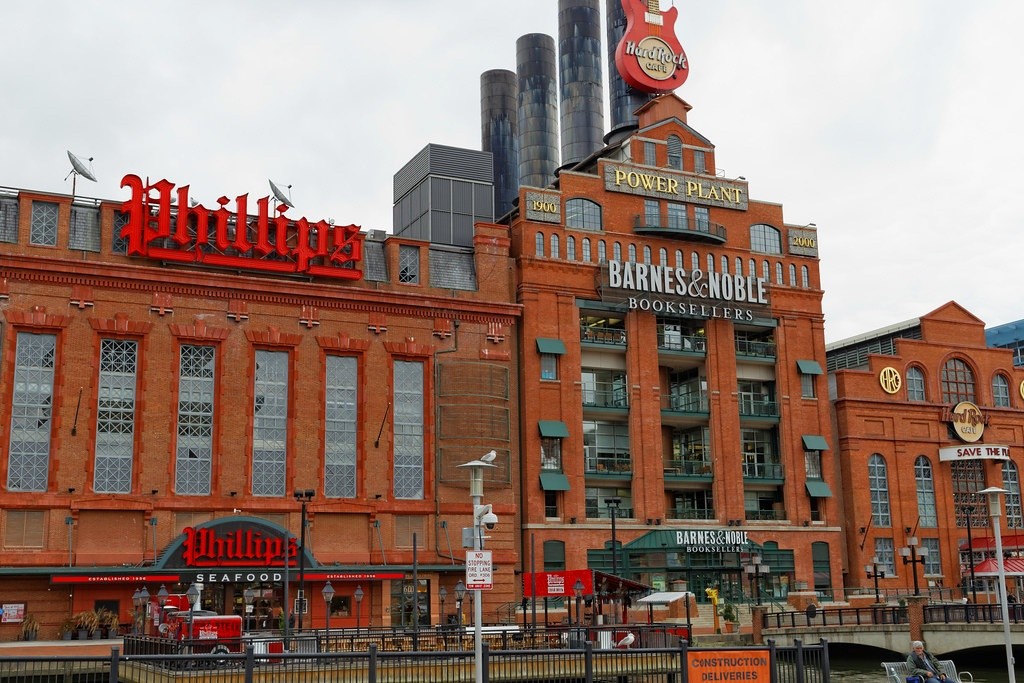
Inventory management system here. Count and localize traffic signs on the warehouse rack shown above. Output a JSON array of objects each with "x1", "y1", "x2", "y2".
[{"x1": 465, "y1": 550, "x2": 494, "y2": 591}]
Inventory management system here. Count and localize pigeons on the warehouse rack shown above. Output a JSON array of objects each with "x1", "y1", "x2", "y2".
[{"x1": 617, "y1": 633, "x2": 635, "y2": 649}]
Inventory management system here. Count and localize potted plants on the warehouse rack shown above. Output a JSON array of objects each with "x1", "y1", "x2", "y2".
[
  {"x1": 18, "y1": 613, "x2": 40, "y2": 641},
  {"x1": 723, "y1": 605, "x2": 735, "y2": 633},
  {"x1": 897, "y1": 599, "x2": 907, "y2": 623},
  {"x1": 61, "y1": 607, "x2": 118, "y2": 640}
]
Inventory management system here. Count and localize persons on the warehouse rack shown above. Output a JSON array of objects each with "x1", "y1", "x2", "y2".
[
  {"x1": 271, "y1": 601, "x2": 282, "y2": 629},
  {"x1": 907, "y1": 641, "x2": 955, "y2": 683},
  {"x1": 964, "y1": 594, "x2": 972, "y2": 604}
]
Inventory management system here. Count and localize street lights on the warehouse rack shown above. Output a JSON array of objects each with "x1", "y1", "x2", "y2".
[
  {"x1": 185, "y1": 582, "x2": 201, "y2": 669},
  {"x1": 138, "y1": 585, "x2": 151, "y2": 640},
  {"x1": 132, "y1": 588, "x2": 142, "y2": 638},
  {"x1": 572, "y1": 579, "x2": 586, "y2": 649},
  {"x1": 603, "y1": 497, "x2": 622, "y2": 624},
  {"x1": 452, "y1": 579, "x2": 467, "y2": 659},
  {"x1": 293, "y1": 487, "x2": 316, "y2": 635},
  {"x1": 466, "y1": 590, "x2": 475, "y2": 627},
  {"x1": 244, "y1": 587, "x2": 256, "y2": 645},
  {"x1": 456, "y1": 460, "x2": 500, "y2": 683},
  {"x1": 974, "y1": 486, "x2": 1016, "y2": 683},
  {"x1": 438, "y1": 586, "x2": 448, "y2": 637},
  {"x1": 961, "y1": 505, "x2": 979, "y2": 622},
  {"x1": 897, "y1": 537, "x2": 928, "y2": 596},
  {"x1": 743, "y1": 556, "x2": 770, "y2": 606},
  {"x1": 863, "y1": 556, "x2": 887, "y2": 603},
  {"x1": 321, "y1": 579, "x2": 335, "y2": 664},
  {"x1": 157, "y1": 582, "x2": 169, "y2": 643},
  {"x1": 353, "y1": 585, "x2": 364, "y2": 648}
]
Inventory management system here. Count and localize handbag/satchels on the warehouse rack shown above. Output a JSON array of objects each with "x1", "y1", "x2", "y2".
[{"x1": 906, "y1": 676, "x2": 921, "y2": 683}]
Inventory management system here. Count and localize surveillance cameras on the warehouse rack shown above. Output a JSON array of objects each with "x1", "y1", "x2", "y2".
[{"x1": 481, "y1": 513, "x2": 498, "y2": 530}]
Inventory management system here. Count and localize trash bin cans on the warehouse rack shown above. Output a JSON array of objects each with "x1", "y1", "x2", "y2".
[
  {"x1": 294, "y1": 634, "x2": 318, "y2": 662},
  {"x1": 570, "y1": 628, "x2": 586, "y2": 649}
]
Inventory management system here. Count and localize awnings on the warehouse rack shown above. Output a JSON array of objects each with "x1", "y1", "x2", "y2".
[
  {"x1": 536, "y1": 337, "x2": 566, "y2": 355},
  {"x1": 805, "y1": 481, "x2": 831, "y2": 498},
  {"x1": 802, "y1": 435, "x2": 829, "y2": 451},
  {"x1": 960, "y1": 535, "x2": 1024, "y2": 552},
  {"x1": 539, "y1": 474, "x2": 570, "y2": 491},
  {"x1": 538, "y1": 420, "x2": 570, "y2": 438},
  {"x1": 797, "y1": 359, "x2": 824, "y2": 374}
]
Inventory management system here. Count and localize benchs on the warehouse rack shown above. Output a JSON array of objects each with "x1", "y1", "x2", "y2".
[{"x1": 881, "y1": 660, "x2": 974, "y2": 683}]
